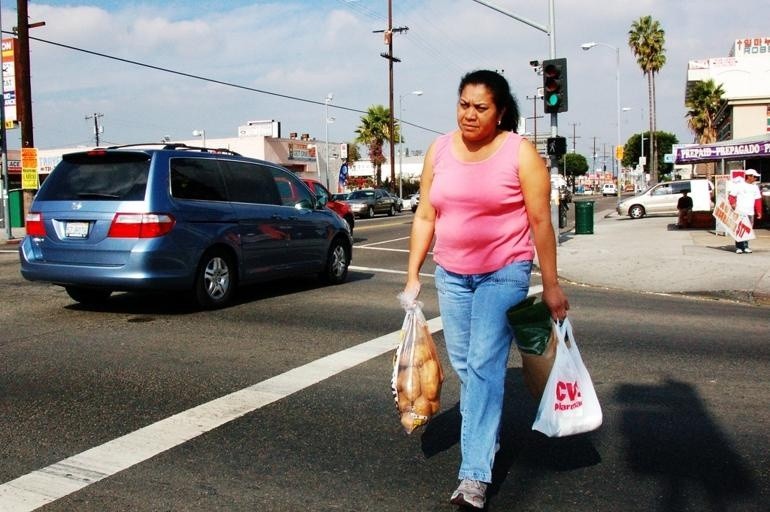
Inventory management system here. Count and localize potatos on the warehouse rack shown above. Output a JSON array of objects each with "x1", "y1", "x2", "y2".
[{"x1": 397, "y1": 324, "x2": 444, "y2": 431}]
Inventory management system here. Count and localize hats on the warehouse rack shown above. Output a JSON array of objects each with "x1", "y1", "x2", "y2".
[{"x1": 745, "y1": 168, "x2": 761, "y2": 177}]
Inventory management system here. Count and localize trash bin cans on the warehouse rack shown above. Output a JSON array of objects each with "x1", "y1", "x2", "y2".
[{"x1": 573, "y1": 201, "x2": 595, "y2": 235}]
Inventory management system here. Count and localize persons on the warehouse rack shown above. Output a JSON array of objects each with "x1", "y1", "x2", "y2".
[
  {"x1": 728, "y1": 168, "x2": 764, "y2": 254},
  {"x1": 676, "y1": 190, "x2": 694, "y2": 227},
  {"x1": 402, "y1": 67, "x2": 573, "y2": 507}
]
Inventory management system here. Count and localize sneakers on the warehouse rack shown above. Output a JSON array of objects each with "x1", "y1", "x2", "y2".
[
  {"x1": 736, "y1": 249, "x2": 743, "y2": 254},
  {"x1": 744, "y1": 248, "x2": 752, "y2": 253},
  {"x1": 450, "y1": 479, "x2": 487, "y2": 509}
]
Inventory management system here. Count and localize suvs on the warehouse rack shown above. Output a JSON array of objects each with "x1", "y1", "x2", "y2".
[{"x1": 274, "y1": 178, "x2": 357, "y2": 237}]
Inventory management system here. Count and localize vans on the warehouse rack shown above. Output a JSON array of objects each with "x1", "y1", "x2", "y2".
[
  {"x1": 602, "y1": 183, "x2": 619, "y2": 196},
  {"x1": 15, "y1": 143, "x2": 357, "y2": 302},
  {"x1": 617, "y1": 179, "x2": 715, "y2": 219}
]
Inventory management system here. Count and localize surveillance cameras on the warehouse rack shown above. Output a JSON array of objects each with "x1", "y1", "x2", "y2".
[{"x1": 529, "y1": 60, "x2": 543, "y2": 68}]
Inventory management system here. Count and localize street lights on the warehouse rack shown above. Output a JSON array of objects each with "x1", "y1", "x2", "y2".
[
  {"x1": 306, "y1": 144, "x2": 321, "y2": 181},
  {"x1": 622, "y1": 107, "x2": 651, "y2": 173},
  {"x1": 325, "y1": 92, "x2": 336, "y2": 193},
  {"x1": 193, "y1": 129, "x2": 206, "y2": 150},
  {"x1": 581, "y1": 41, "x2": 622, "y2": 205},
  {"x1": 398, "y1": 90, "x2": 425, "y2": 206},
  {"x1": 568, "y1": 148, "x2": 618, "y2": 190}
]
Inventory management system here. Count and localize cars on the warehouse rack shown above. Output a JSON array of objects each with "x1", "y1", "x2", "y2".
[
  {"x1": 559, "y1": 189, "x2": 573, "y2": 228},
  {"x1": 333, "y1": 189, "x2": 404, "y2": 221},
  {"x1": 410, "y1": 189, "x2": 421, "y2": 213}
]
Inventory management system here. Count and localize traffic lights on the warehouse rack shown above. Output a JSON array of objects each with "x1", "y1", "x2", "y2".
[
  {"x1": 544, "y1": 64, "x2": 564, "y2": 108},
  {"x1": 548, "y1": 137, "x2": 566, "y2": 155}
]
